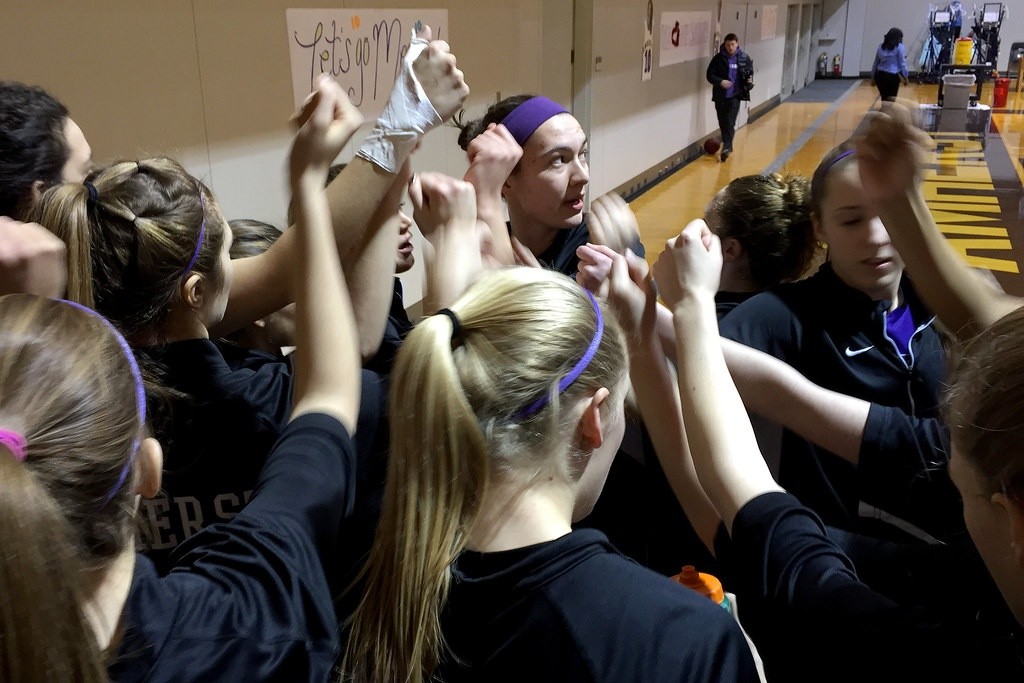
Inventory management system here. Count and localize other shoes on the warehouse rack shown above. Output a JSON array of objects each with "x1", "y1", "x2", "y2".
[{"x1": 721, "y1": 144, "x2": 732, "y2": 161}]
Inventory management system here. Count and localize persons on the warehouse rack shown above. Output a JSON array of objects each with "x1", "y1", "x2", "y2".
[
  {"x1": 0, "y1": 24, "x2": 1024, "y2": 683},
  {"x1": 707, "y1": 33, "x2": 753, "y2": 161}
]
